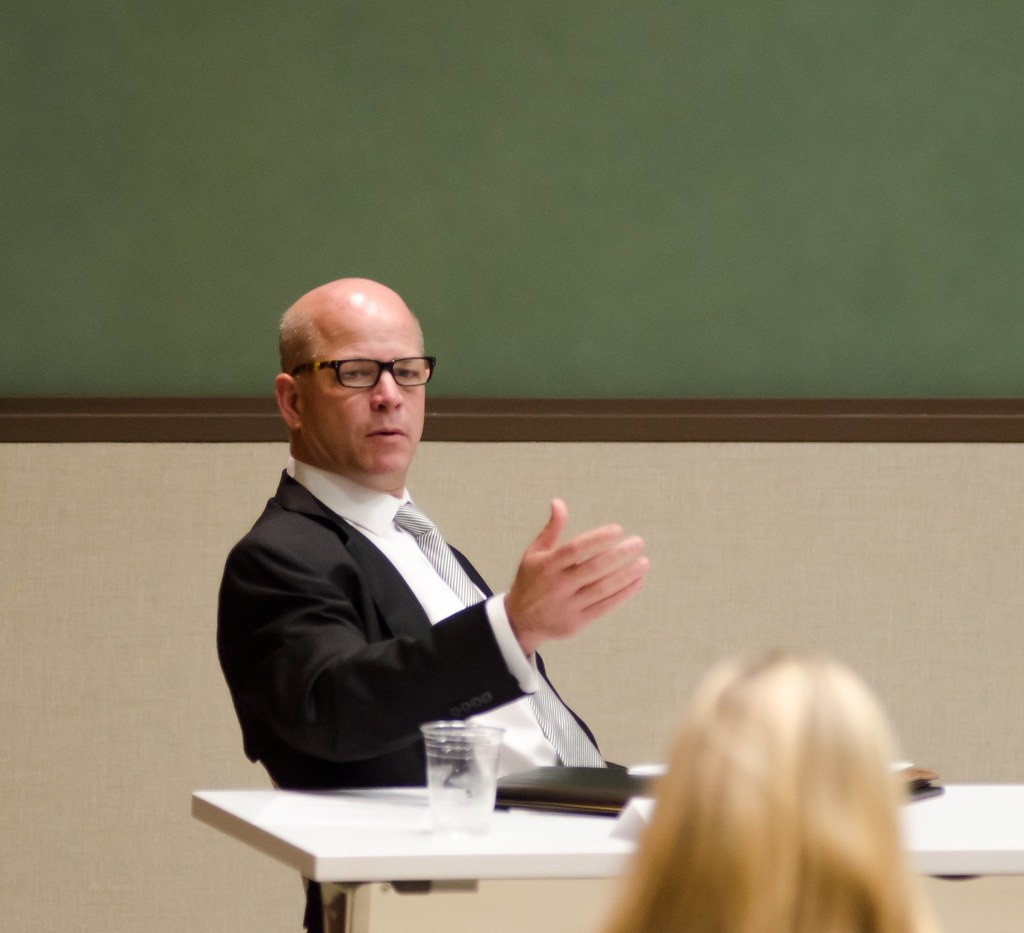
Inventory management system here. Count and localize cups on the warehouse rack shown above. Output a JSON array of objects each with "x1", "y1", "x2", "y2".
[{"x1": 420, "y1": 722, "x2": 506, "y2": 832}]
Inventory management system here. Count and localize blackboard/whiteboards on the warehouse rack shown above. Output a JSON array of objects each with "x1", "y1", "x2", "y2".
[{"x1": 1, "y1": 0, "x2": 1024, "y2": 447}]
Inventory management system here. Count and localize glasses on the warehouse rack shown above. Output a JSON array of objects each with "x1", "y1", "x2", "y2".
[{"x1": 291, "y1": 357, "x2": 436, "y2": 389}]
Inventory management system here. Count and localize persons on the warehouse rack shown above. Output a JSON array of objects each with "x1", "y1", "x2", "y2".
[
  {"x1": 601, "y1": 643, "x2": 940, "y2": 933},
  {"x1": 216, "y1": 277, "x2": 650, "y2": 933}
]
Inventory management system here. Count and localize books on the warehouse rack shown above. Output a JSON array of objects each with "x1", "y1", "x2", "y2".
[
  {"x1": 898, "y1": 767, "x2": 944, "y2": 801},
  {"x1": 495, "y1": 760, "x2": 656, "y2": 816}
]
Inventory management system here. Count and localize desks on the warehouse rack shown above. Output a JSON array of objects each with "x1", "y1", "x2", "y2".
[{"x1": 193, "y1": 785, "x2": 1024, "y2": 933}]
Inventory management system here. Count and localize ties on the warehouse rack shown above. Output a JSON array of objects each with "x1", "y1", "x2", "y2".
[{"x1": 397, "y1": 506, "x2": 607, "y2": 768}]
